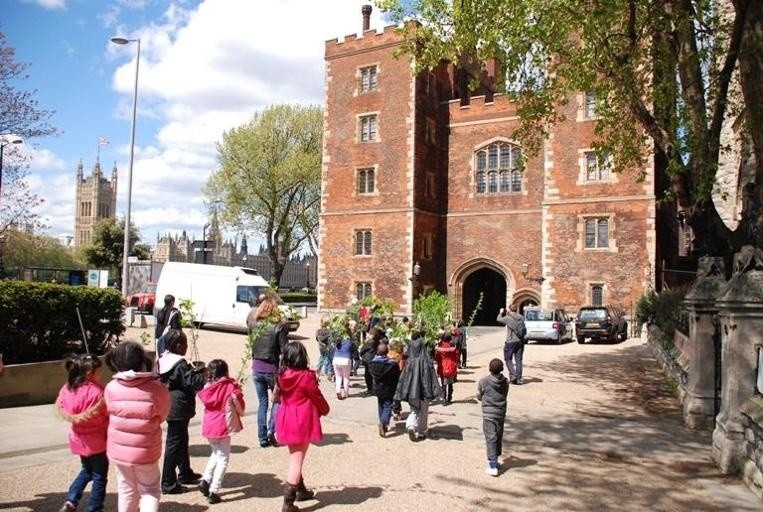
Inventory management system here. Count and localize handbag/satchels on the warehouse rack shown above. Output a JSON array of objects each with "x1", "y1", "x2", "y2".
[{"x1": 157, "y1": 325, "x2": 171, "y2": 358}]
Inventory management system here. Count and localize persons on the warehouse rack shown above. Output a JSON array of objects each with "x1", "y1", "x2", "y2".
[
  {"x1": 476, "y1": 356, "x2": 510, "y2": 477},
  {"x1": 269, "y1": 341, "x2": 330, "y2": 512},
  {"x1": 156, "y1": 294, "x2": 183, "y2": 359},
  {"x1": 496, "y1": 303, "x2": 527, "y2": 385},
  {"x1": 313, "y1": 320, "x2": 467, "y2": 442},
  {"x1": 246, "y1": 293, "x2": 290, "y2": 448},
  {"x1": 198, "y1": 359, "x2": 245, "y2": 504},
  {"x1": 0, "y1": 353, "x2": 4, "y2": 376},
  {"x1": 55, "y1": 353, "x2": 109, "y2": 511},
  {"x1": 153, "y1": 331, "x2": 215, "y2": 494},
  {"x1": 102, "y1": 339, "x2": 173, "y2": 512}
]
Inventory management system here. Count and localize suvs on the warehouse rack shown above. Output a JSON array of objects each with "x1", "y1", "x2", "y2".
[{"x1": 126, "y1": 281, "x2": 159, "y2": 314}]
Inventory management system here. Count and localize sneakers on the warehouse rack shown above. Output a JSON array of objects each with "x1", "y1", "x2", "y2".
[
  {"x1": 60, "y1": 431, "x2": 314, "y2": 511},
  {"x1": 487, "y1": 468, "x2": 498, "y2": 476},
  {"x1": 379, "y1": 413, "x2": 426, "y2": 441}
]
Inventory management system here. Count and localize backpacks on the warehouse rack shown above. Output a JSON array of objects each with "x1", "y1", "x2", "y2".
[{"x1": 512, "y1": 320, "x2": 526, "y2": 338}]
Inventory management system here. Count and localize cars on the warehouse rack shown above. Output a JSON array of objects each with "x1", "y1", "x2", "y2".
[{"x1": 522, "y1": 307, "x2": 573, "y2": 344}]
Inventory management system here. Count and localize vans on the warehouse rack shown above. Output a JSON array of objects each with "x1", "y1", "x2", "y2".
[{"x1": 574, "y1": 305, "x2": 627, "y2": 344}]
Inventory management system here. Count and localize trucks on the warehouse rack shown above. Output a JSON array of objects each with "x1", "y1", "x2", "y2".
[{"x1": 153, "y1": 261, "x2": 299, "y2": 336}]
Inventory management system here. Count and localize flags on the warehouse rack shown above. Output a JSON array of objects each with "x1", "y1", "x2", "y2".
[
  {"x1": 360, "y1": 305, "x2": 369, "y2": 318},
  {"x1": 99, "y1": 135, "x2": 109, "y2": 147}
]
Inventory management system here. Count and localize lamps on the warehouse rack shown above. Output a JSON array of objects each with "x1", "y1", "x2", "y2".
[
  {"x1": 521, "y1": 261, "x2": 546, "y2": 284},
  {"x1": 408, "y1": 261, "x2": 423, "y2": 281}
]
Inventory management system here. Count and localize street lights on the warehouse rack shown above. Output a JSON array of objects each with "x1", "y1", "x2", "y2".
[
  {"x1": 241, "y1": 255, "x2": 247, "y2": 267},
  {"x1": 305, "y1": 260, "x2": 309, "y2": 287},
  {"x1": 0, "y1": 140, "x2": 23, "y2": 193},
  {"x1": 110, "y1": 35, "x2": 141, "y2": 304},
  {"x1": 200, "y1": 221, "x2": 210, "y2": 241}
]
